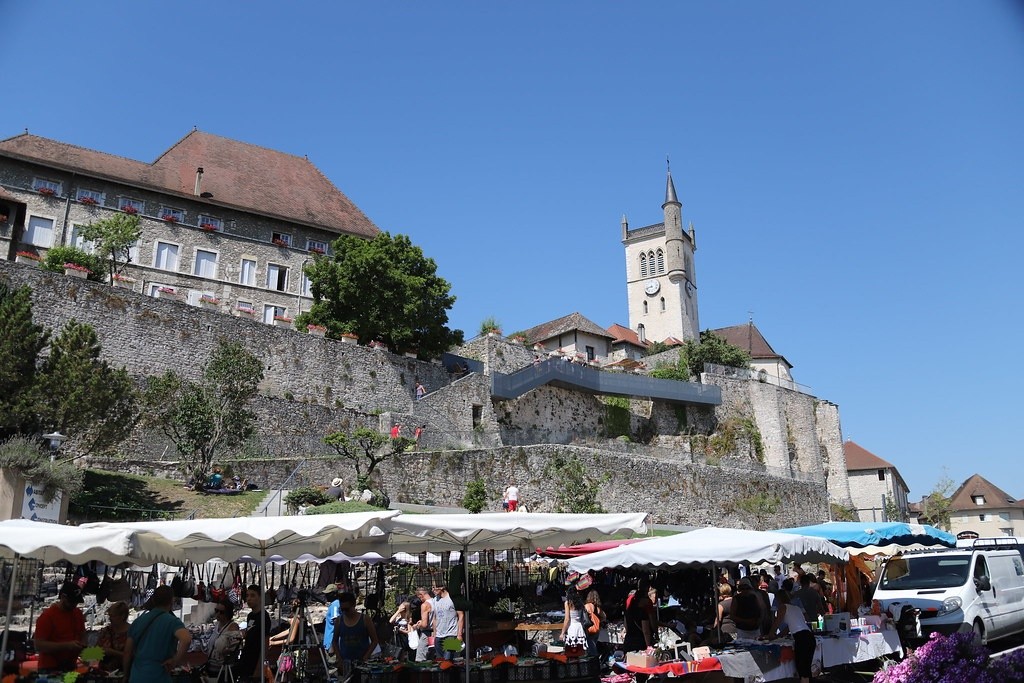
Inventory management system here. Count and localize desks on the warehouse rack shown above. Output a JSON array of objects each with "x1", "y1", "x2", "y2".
[
  {"x1": 514, "y1": 623, "x2": 564, "y2": 640},
  {"x1": 614, "y1": 629, "x2": 904, "y2": 683}
]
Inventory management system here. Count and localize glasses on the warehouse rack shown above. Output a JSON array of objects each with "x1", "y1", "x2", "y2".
[
  {"x1": 214, "y1": 608, "x2": 225, "y2": 615},
  {"x1": 341, "y1": 604, "x2": 353, "y2": 612}
]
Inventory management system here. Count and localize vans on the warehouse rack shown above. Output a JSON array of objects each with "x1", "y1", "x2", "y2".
[{"x1": 871, "y1": 537, "x2": 1024, "y2": 645}]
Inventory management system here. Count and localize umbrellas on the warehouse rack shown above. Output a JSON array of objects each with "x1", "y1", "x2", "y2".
[{"x1": 0, "y1": 511, "x2": 957, "y2": 673}]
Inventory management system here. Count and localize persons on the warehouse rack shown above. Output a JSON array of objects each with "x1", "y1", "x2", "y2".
[
  {"x1": 391, "y1": 423, "x2": 400, "y2": 437},
  {"x1": 584, "y1": 590, "x2": 606, "y2": 654},
  {"x1": 207, "y1": 468, "x2": 223, "y2": 489},
  {"x1": 502, "y1": 482, "x2": 519, "y2": 512},
  {"x1": 239, "y1": 585, "x2": 271, "y2": 683},
  {"x1": 626, "y1": 577, "x2": 658, "y2": 633},
  {"x1": 414, "y1": 425, "x2": 421, "y2": 450},
  {"x1": 34, "y1": 581, "x2": 88, "y2": 677},
  {"x1": 189, "y1": 599, "x2": 241, "y2": 683},
  {"x1": 416, "y1": 381, "x2": 426, "y2": 399},
  {"x1": 331, "y1": 581, "x2": 464, "y2": 683},
  {"x1": 96, "y1": 601, "x2": 132, "y2": 672},
  {"x1": 622, "y1": 592, "x2": 655, "y2": 683},
  {"x1": 322, "y1": 583, "x2": 341, "y2": 683},
  {"x1": 559, "y1": 585, "x2": 589, "y2": 651},
  {"x1": 123, "y1": 585, "x2": 192, "y2": 683},
  {"x1": 324, "y1": 478, "x2": 345, "y2": 502},
  {"x1": 713, "y1": 562, "x2": 829, "y2": 683}
]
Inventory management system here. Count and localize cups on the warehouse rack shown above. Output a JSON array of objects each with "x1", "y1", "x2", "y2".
[
  {"x1": 850, "y1": 618, "x2": 866, "y2": 627},
  {"x1": 811, "y1": 622, "x2": 817, "y2": 630}
]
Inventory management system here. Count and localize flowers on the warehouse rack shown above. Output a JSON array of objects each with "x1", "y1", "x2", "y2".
[
  {"x1": 155, "y1": 286, "x2": 176, "y2": 294},
  {"x1": 200, "y1": 295, "x2": 417, "y2": 356},
  {"x1": 64, "y1": 263, "x2": 91, "y2": 274},
  {"x1": 39, "y1": 186, "x2": 325, "y2": 256},
  {"x1": 114, "y1": 274, "x2": 135, "y2": 283},
  {"x1": 16, "y1": 250, "x2": 41, "y2": 260}
]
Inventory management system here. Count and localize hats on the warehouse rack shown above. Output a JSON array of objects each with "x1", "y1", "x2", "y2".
[
  {"x1": 322, "y1": 584, "x2": 338, "y2": 593},
  {"x1": 332, "y1": 478, "x2": 343, "y2": 487},
  {"x1": 61, "y1": 582, "x2": 84, "y2": 603},
  {"x1": 737, "y1": 578, "x2": 753, "y2": 592}
]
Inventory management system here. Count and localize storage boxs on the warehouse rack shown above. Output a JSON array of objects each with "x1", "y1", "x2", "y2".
[{"x1": 352, "y1": 656, "x2": 601, "y2": 683}]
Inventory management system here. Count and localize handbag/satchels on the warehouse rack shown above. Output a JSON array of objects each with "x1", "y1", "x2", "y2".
[
  {"x1": 580, "y1": 605, "x2": 594, "y2": 630},
  {"x1": 277, "y1": 654, "x2": 294, "y2": 674},
  {"x1": 408, "y1": 627, "x2": 419, "y2": 650},
  {"x1": 63, "y1": 560, "x2": 386, "y2": 609},
  {"x1": 587, "y1": 603, "x2": 601, "y2": 633},
  {"x1": 207, "y1": 659, "x2": 238, "y2": 672}
]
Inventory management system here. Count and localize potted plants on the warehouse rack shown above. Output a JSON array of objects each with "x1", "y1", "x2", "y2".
[{"x1": 490, "y1": 598, "x2": 516, "y2": 629}]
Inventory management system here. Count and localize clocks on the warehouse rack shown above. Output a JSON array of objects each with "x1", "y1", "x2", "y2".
[{"x1": 644, "y1": 278, "x2": 660, "y2": 295}]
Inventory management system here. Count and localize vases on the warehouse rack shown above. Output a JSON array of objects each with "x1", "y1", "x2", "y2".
[
  {"x1": 404, "y1": 351, "x2": 417, "y2": 359},
  {"x1": 430, "y1": 357, "x2": 442, "y2": 364},
  {"x1": 65, "y1": 268, "x2": 88, "y2": 279},
  {"x1": 373, "y1": 345, "x2": 388, "y2": 352},
  {"x1": 274, "y1": 320, "x2": 292, "y2": 330},
  {"x1": 236, "y1": 310, "x2": 255, "y2": 321},
  {"x1": 202, "y1": 302, "x2": 217, "y2": 311},
  {"x1": 113, "y1": 281, "x2": 135, "y2": 290},
  {"x1": 155, "y1": 292, "x2": 174, "y2": 300},
  {"x1": 310, "y1": 328, "x2": 326, "y2": 338},
  {"x1": 342, "y1": 337, "x2": 358, "y2": 347},
  {"x1": 15, "y1": 256, "x2": 40, "y2": 267}
]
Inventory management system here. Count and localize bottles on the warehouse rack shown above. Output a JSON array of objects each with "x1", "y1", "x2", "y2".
[{"x1": 818, "y1": 615, "x2": 823, "y2": 631}]
